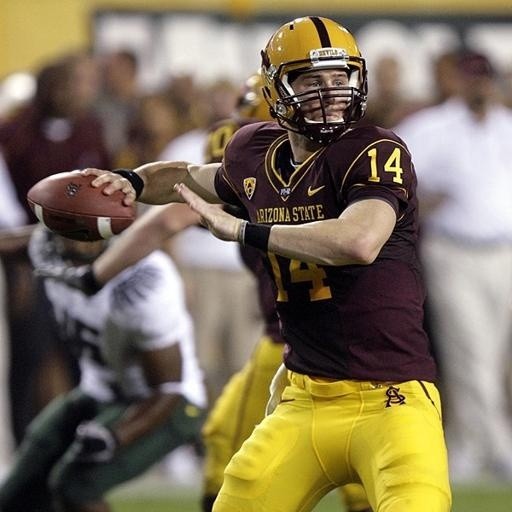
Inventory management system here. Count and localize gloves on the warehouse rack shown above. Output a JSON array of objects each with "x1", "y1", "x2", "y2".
[{"x1": 75, "y1": 420, "x2": 117, "y2": 467}]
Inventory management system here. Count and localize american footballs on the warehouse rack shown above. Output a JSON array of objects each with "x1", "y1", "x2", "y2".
[{"x1": 27, "y1": 172, "x2": 138, "y2": 242}]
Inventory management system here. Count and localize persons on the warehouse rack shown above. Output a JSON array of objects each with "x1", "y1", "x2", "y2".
[
  {"x1": 34, "y1": 68, "x2": 374, "y2": 512},
  {"x1": 1, "y1": 46, "x2": 239, "y2": 230},
  {"x1": 212, "y1": 16, "x2": 454, "y2": 512},
  {"x1": 0, "y1": 213, "x2": 208, "y2": 512},
  {"x1": 370, "y1": 46, "x2": 512, "y2": 228}
]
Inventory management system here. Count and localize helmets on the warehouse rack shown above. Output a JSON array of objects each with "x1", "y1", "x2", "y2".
[{"x1": 260, "y1": 15, "x2": 364, "y2": 121}]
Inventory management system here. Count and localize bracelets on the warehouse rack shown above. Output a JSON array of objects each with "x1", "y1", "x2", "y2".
[
  {"x1": 245, "y1": 222, "x2": 272, "y2": 251},
  {"x1": 239, "y1": 220, "x2": 249, "y2": 244}
]
